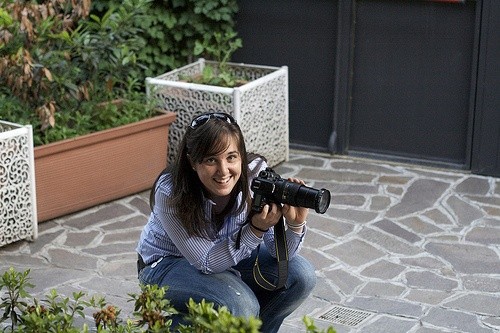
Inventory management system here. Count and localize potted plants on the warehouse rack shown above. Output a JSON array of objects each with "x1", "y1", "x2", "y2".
[
  {"x1": 144, "y1": 0, "x2": 289, "y2": 168},
  {"x1": 0, "y1": 0, "x2": 179, "y2": 221}
]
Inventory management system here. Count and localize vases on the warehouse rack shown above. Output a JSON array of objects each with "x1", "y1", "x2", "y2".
[{"x1": 0, "y1": 120, "x2": 39, "y2": 247}]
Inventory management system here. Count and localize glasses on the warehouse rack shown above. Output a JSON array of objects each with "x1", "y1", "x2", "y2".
[{"x1": 190, "y1": 113, "x2": 232, "y2": 130}]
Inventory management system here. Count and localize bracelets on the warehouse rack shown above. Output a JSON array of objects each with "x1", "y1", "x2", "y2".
[
  {"x1": 285, "y1": 220, "x2": 307, "y2": 228},
  {"x1": 250, "y1": 220, "x2": 269, "y2": 233}
]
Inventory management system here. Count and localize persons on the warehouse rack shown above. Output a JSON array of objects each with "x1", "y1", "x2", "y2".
[{"x1": 134, "y1": 112, "x2": 316, "y2": 333}]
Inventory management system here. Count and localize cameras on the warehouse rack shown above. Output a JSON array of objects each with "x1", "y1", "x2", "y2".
[{"x1": 250, "y1": 167, "x2": 331, "y2": 215}]
